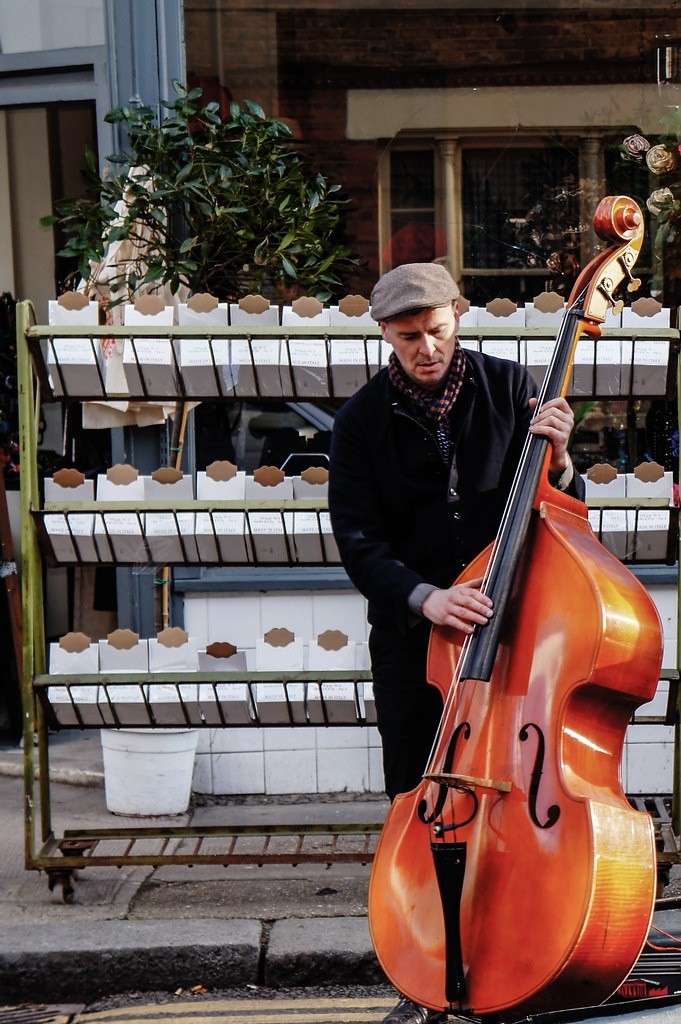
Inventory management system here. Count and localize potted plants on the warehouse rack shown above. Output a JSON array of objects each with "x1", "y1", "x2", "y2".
[{"x1": 39, "y1": 80, "x2": 361, "y2": 819}]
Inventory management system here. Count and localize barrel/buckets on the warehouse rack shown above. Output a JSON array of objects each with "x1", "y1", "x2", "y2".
[{"x1": 101, "y1": 728, "x2": 197, "y2": 817}]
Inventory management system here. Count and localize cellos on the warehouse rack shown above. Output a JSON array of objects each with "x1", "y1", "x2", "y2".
[{"x1": 367, "y1": 195, "x2": 666, "y2": 1018}]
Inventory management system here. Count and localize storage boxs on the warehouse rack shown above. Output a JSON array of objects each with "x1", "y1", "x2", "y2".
[{"x1": 40, "y1": 290, "x2": 677, "y2": 726}]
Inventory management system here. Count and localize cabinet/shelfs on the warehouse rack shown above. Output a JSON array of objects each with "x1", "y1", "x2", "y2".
[{"x1": 15, "y1": 301, "x2": 680, "y2": 871}]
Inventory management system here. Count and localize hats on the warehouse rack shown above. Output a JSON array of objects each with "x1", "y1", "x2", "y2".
[{"x1": 370, "y1": 262, "x2": 460, "y2": 322}]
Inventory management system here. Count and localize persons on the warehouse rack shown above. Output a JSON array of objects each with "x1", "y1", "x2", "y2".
[
  {"x1": 389, "y1": 189, "x2": 454, "y2": 279},
  {"x1": 327, "y1": 262, "x2": 588, "y2": 1024}
]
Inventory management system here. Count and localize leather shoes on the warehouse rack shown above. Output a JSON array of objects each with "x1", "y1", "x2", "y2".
[{"x1": 381, "y1": 993, "x2": 448, "y2": 1024}]
